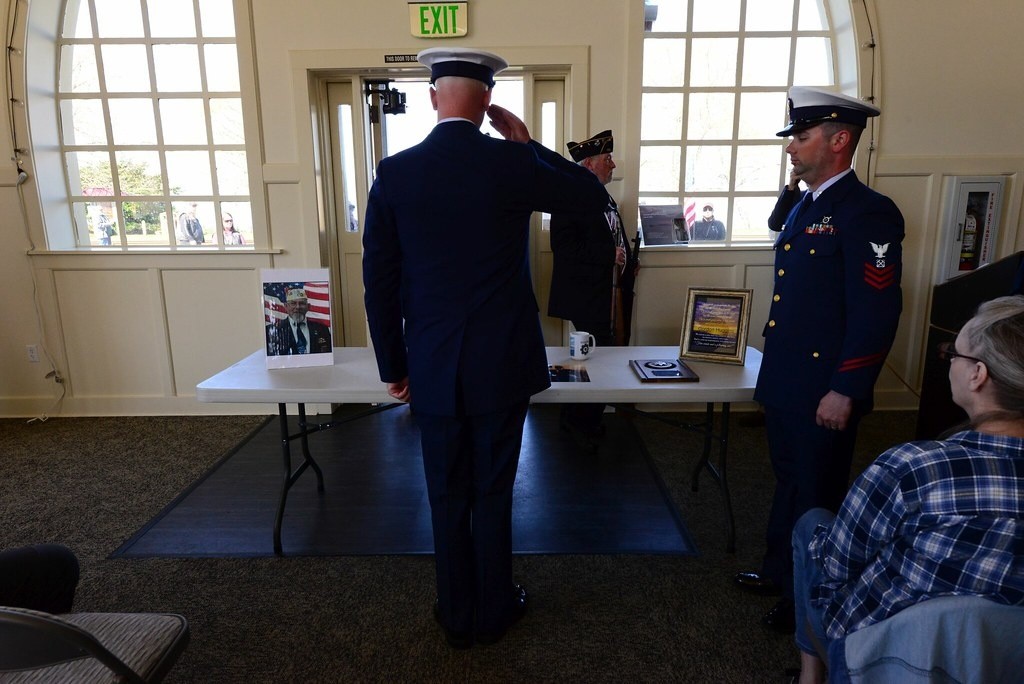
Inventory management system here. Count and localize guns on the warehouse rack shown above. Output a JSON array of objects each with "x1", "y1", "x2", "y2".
[
  {"x1": 607, "y1": 227, "x2": 627, "y2": 346},
  {"x1": 622, "y1": 230, "x2": 642, "y2": 346}
]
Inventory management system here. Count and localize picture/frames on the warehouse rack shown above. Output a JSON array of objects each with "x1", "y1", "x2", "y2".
[
  {"x1": 262, "y1": 280, "x2": 332, "y2": 356},
  {"x1": 677, "y1": 286, "x2": 754, "y2": 365}
]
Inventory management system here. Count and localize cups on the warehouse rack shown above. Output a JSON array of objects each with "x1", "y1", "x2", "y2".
[{"x1": 569, "y1": 331, "x2": 595, "y2": 361}]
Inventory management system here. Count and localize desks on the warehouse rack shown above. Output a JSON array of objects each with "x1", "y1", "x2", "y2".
[{"x1": 195, "y1": 337, "x2": 770, "y2": 555}]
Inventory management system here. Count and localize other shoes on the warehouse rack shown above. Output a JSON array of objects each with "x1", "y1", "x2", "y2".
[{"x1": 558, "y1": 420, "x2": 605, "y2": 450}]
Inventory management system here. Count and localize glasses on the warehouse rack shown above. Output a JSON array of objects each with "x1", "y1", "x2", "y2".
[
  {"x1": 940, "y1": 340, "x2": 992, "y2": 380},
  {"x1": 703, "y1": 207, "x2": 713, "y2": 212}
]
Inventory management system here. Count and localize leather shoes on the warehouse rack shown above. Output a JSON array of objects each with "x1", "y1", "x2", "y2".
[
  {"x1": 734, "y1": 564, "x2": 774, "y2": 587},
  {"x1": 762, "y1": 597, "x2": 796, "y2": 634},
  {"x1": 433, "y1": 584, "x2": 529, "y2": 649}
]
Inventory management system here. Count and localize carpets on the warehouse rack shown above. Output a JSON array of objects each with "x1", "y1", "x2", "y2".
[{"x1": 0, "y1": 409, "x2": 972, "y2": 684}]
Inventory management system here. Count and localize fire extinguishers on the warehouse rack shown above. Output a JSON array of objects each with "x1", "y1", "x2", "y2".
[{"x1": 959, "y1": 200, "x2": 978, "y2": 270}]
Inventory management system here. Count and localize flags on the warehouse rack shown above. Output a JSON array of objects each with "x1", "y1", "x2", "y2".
[
  {"x1": 264, "y1": 282, "x2": 330, "y2": 326},
  {"x1": 684, "y1": 198, "x2": 696, "y2": 226}
]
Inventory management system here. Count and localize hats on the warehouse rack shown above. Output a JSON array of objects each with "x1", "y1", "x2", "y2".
[
  {"x1": 567, "y1": 129, "x2": 615, "y2": 164},
  {"x1": 776, "y1": 85, "x2": 880, "y2": 137},
  {"x1": 285, "y1": 289, "x2": 308, "y2": 301},
  {"x1": 417, "y1": 45, "x2": 509, "y2": 88},
  {"x1": 702, "y1": 203, "x2": 714, "y2": 209}
]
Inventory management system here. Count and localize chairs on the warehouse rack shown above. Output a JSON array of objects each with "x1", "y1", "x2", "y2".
[
  {"x1": 0, "y1": 603, "x2": 188, "y2": 684},
  {"x1": 805, "y1": 593, "x2": 1024, "y2": 684}
]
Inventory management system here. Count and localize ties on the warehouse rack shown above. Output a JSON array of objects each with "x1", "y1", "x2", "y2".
[
  {"x1": 792, "y1": 192, "x2": 815, "y2": 230},
  {"x1": 292, "y1": 321, "x2": 308, "y2": 354}
]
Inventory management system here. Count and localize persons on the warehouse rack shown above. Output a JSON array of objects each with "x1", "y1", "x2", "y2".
[
  {"x1": 213, "y1": 213, "x2": 246, "y2": 245},
  {"x1": 94, "y1": 206, "x2": 118, "y2": 244},
  {"x1": 266, "y1": 290, "x2": 332, "y2": 356},
  {"x1": 362, "y1": 48, "x2": 610, "y2": 650},
  {"x1": 689, "y1": 201, "x2": 725, "y2": 241},
  {"x1": 736, "y1": 85, "x2": 906, "y2": 633},
  {"x1": 548, "y1": 129, "x2": 641, "y2": 450},
  {"x1": 183, "y1": 206, "x2": 205, "y2": 245},
  {"x1": 793, "y1": 298, "x2": 1024, "y2": 684}
]
